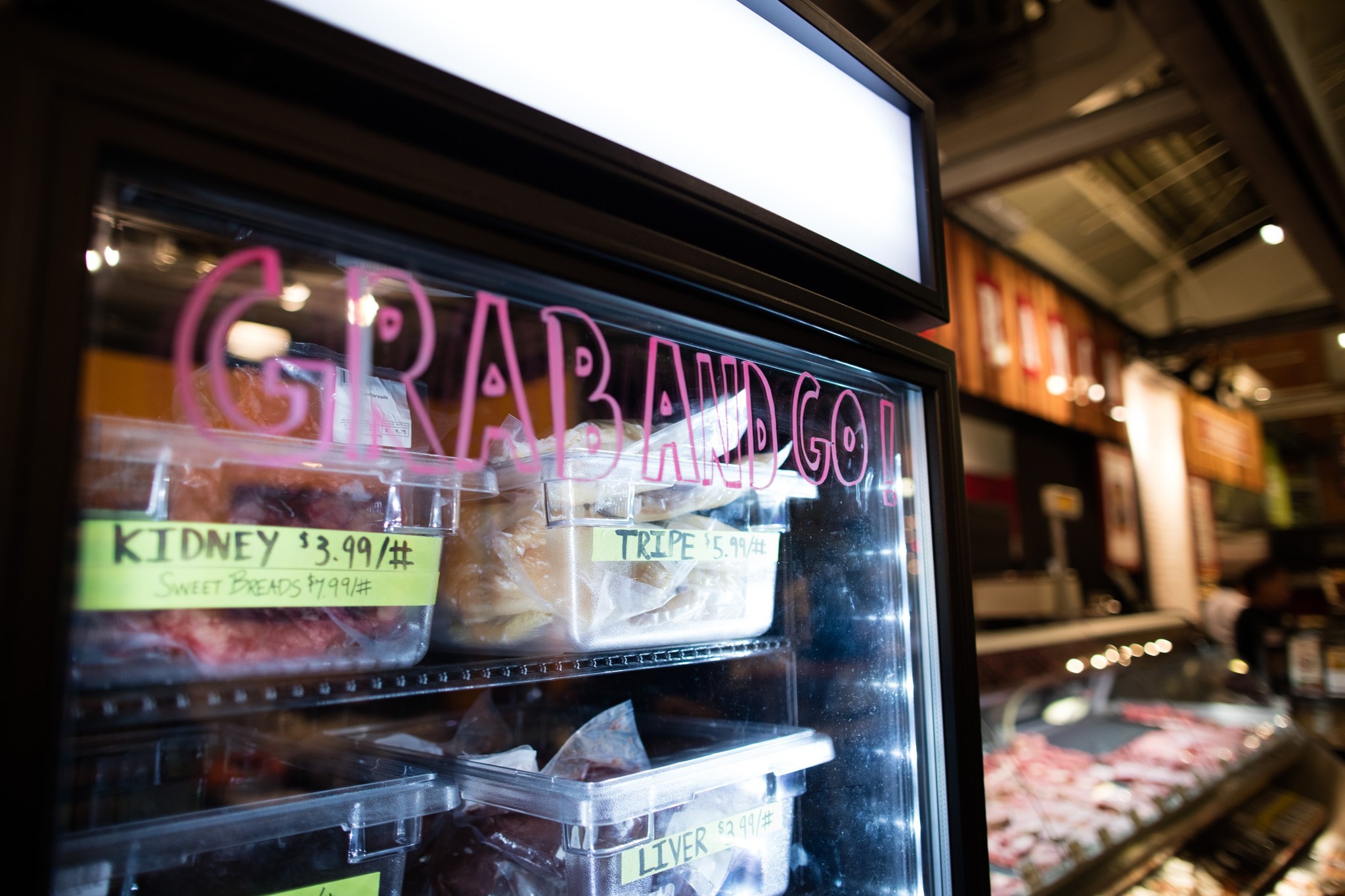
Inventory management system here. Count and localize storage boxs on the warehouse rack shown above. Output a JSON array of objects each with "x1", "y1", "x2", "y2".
[
  {"x1": 64, "y1": 405, "x2": 825, "y2": 696},
  {"x1": 30, "y1": 718, "x2": 839, "y2": 896}
]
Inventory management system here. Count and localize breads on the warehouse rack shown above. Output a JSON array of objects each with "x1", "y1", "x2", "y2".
[{"x1": 433, "y1": 417, "x2": 773, "y2": 650}]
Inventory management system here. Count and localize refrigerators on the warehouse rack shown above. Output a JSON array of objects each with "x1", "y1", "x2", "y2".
[{"x1": 0, "y1": 0, "x2": 995, "y2": 895}]
